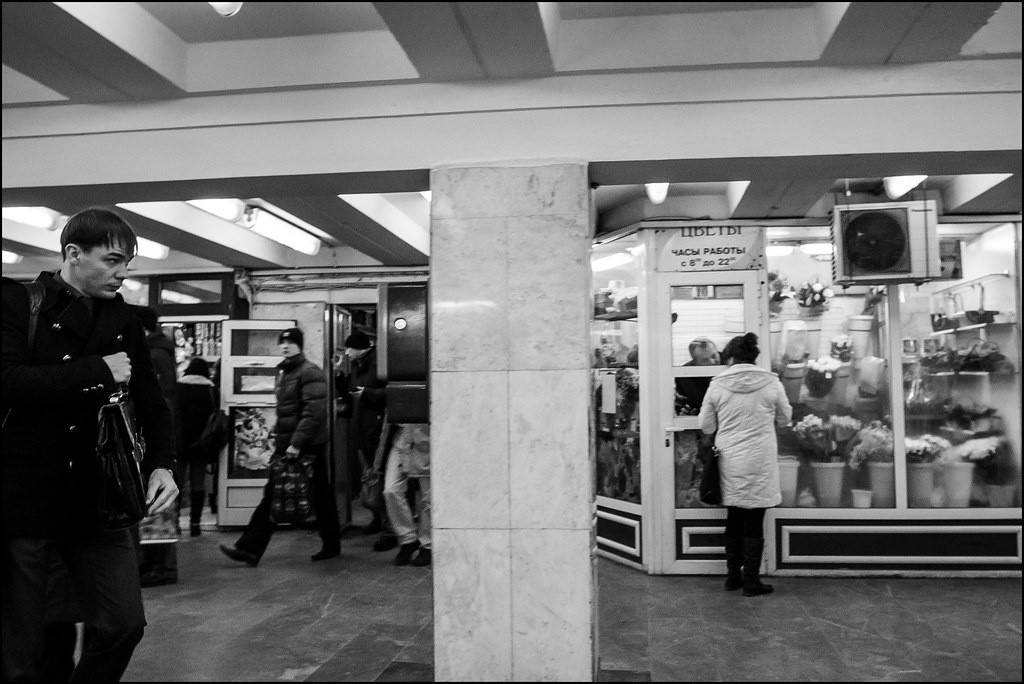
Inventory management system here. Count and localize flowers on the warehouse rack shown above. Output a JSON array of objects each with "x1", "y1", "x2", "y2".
[
  {"x1": 804, "y1": 334, "x2": 854, "y2": 399},
  {"x1": 792, "y1": 414, "x2": 1010, "y2": 486},
  {"x1": 766, "y1": 271, "x2": 833, "y2": 307}
]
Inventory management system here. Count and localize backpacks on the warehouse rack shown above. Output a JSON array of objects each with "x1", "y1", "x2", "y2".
[{"x1": 190, "y1": 386, "x2": 230, "y2": 464}]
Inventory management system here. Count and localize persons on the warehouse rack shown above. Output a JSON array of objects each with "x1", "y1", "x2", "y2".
[
  {"x1": 676, "y1": 338, "x2": 726, "y2": 504},
  {"x1": 700, "y1": 333, "x2": 792, "y2": 596},
  {"x1": 218, "y1": 328, "x2": 341, "y2": 566},
  {"x1": 332, "y1": 331, "x2": 431, "y2": 566},
  {"x1": 0, "y1": 206, "x2": 181, "y2": 683},
  {"x1": 134, "y1": 306, "x2": 220, "y2": 537}
]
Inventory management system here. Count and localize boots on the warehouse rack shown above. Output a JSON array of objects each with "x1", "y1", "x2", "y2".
[
  {"x1": 743, "y1": 537, "x2": 774, "y2": 597},
  {"x1": 724, "y1": 535, "x2": 742, "y2": 591}
]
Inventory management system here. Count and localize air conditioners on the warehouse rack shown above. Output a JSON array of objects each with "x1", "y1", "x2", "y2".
[{"x1": 828, "y1": 199, "x2": 940, "y2": 287}]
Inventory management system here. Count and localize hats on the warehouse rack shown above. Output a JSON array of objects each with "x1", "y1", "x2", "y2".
[
  {"x1": 279, "y1": 328, "x2": 303, "y2": 349},
  {"x1": 344, "y1": 334, "x2": 370, "y2": 350}
]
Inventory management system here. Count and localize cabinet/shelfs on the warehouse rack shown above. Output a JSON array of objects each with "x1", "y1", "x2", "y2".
[
  {"x1": 929, "y1": 273, "x2": 1016, "y2": 376},
  {"x1": 593, "y1": 312, "x2": 641, "y2": 438}
]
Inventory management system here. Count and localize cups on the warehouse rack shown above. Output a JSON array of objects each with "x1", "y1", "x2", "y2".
[
  {"x1": 924, "y1": 339, "x2": 939, "y2": 353},
  {"x1": 852, "y1": 489, "x2": 873, "y2": 508},
  {"x1": 903, "y1": 339, "x2": 917, "y2": 353}
]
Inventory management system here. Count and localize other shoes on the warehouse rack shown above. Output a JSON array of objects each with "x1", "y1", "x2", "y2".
[
  {"x1": 373, "y1": 534, "x2": 397, "y2": 552},
  {"x1": 412, "y1": 547, "x2": 432, "y2": 565},
  {"x1": 190, "y1": 524, "x2": 202, "y2": 537},
  {"x1": 393, "y1": 541, "x2": 420, "y2": 565}
]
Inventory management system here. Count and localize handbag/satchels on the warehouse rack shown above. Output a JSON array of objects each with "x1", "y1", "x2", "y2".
[
  {"x1": 700, "y1": 447, "x2": 722, "y2": 505},
  {"x1": 360, "y1": 467, "x2": 384, "y2": 512},
  {"x1": 267, "y1": 454, "x2": 318, "y2": 524},
  {"x1": 88, "y1": 384, "x2": 146, "y2": 530}
]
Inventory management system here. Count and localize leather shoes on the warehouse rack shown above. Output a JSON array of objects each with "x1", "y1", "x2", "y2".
[
  {"x1": 219, "y1": 544, "x2": 260, "y2": 567},
  {"x1": 310, "y1": 542, "x2": 341, "y2": 561}
]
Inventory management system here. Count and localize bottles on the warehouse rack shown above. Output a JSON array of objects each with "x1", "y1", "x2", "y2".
[{"x1": 195, "y1": 333, "x2": 222, "y2": 356}]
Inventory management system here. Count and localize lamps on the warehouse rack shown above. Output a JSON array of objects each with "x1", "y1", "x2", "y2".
[
  {"x1": 185, "y1": 197, "x2": 322, "y2": 256},
  {"x1": 2, "y1": 206, "x2": 170, "y2": 260}
]
